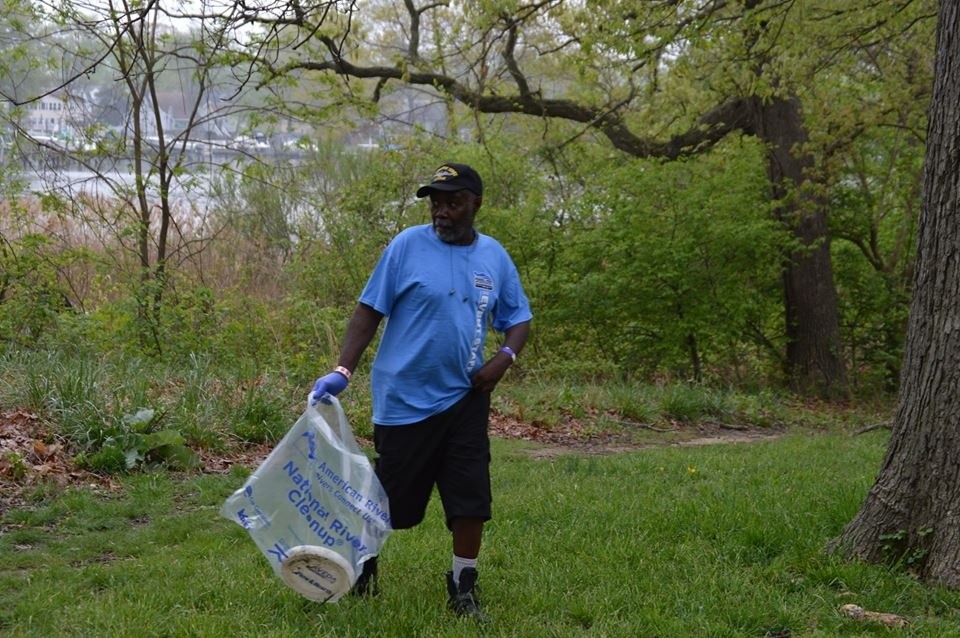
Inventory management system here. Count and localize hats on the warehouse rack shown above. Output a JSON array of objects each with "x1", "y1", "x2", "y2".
[{"x1": 417, "y1": 162, "x2": 485, "y2": 198}]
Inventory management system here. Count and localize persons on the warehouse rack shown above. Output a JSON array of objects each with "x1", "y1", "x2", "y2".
[{"x1": 312, "y1": 163, "x2": 533, "y2": 621}]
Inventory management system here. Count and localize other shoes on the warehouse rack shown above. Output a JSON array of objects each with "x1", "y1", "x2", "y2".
[
  {"x1": 352, "y1": 553, "x2": 383, "y2": 598},
  {"x1": 445, "y1": 566, "x2": 485, "y2": 621}
]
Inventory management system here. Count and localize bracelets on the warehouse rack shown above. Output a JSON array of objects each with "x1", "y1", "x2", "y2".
[
  {"x1": 497, "y1": 346, "x2": 517, "y2": 362},
  {"x1": 334, "y1": 366, "x2": 351, "y2": 382}
]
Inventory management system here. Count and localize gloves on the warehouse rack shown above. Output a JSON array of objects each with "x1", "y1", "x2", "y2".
[{"x1": 310, "y1": 372, "x2": 348, "y2": 406}]
